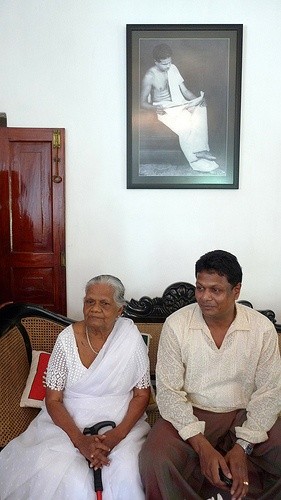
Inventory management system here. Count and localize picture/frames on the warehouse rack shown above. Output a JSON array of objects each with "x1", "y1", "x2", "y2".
[{"x1": 126, "y1": 24, "x2": 243, "y2": 190}]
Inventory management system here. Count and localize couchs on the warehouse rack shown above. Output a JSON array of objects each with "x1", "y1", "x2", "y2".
[{"x1": 0, "y1": 282, "x2": 281, "y2": 500}]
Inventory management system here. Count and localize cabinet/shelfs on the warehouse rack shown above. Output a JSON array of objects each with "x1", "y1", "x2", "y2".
[{"x1": 0, "y1": 127, "x2": 66, "y2": 317}]
